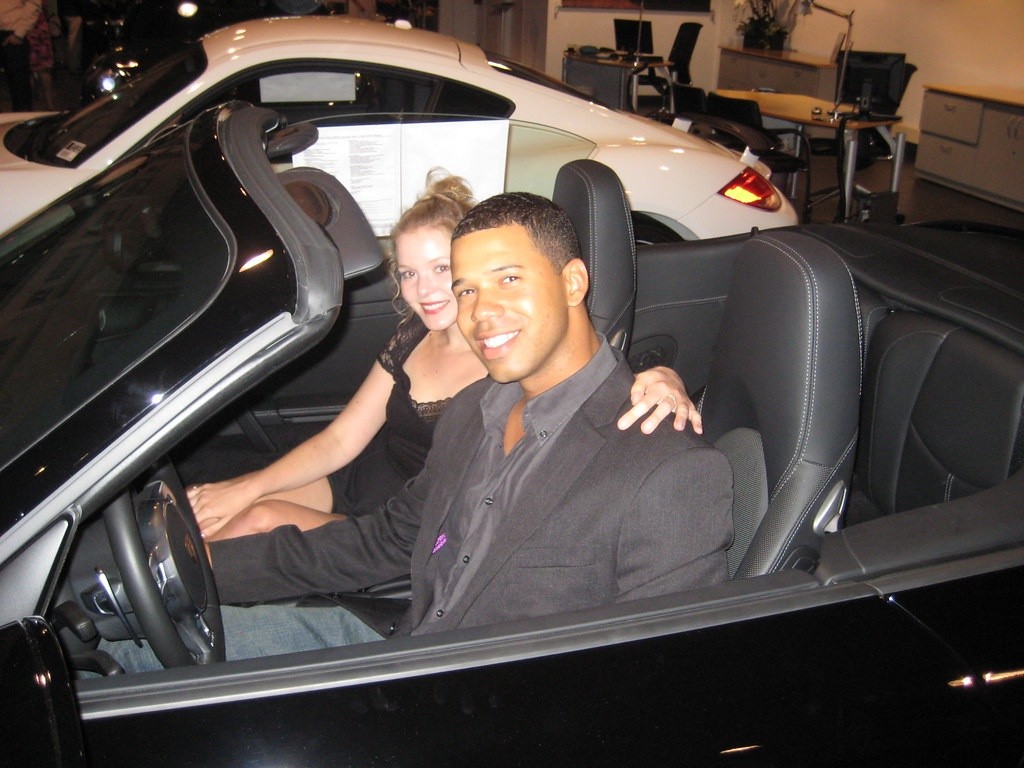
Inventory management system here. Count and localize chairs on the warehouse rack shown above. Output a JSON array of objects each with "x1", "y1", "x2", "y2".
[
  {"x1": 550, "y1": 157, "x2": 638, "y2": 359},
  {"x1": 638, "y1": 21, "x2": 919, "y2": 224},
  {"x1": 695, "y1": 223, "x2": 866, "y2": 578}
]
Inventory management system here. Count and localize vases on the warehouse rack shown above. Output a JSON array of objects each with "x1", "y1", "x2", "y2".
[{"x1": 741, "y1": 23, "x2": 789, "y2": 51}]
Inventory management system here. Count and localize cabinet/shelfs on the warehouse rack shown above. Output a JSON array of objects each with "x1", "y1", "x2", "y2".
[{"x1": 716, "y1": 43, "x2": 838, "y2": 139}]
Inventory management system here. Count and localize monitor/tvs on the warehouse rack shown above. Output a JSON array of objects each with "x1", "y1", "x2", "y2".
[
  {"x1": 835, "y1": 51, "x2": 906, "y2": 121},
  {"x1": 614, "y1": 18, "x2": 653, "y2": 61}
]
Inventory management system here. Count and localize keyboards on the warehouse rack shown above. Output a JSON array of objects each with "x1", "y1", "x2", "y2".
[
  {"x1": 640, "y1": 57, "x2": 663, "y2": 62},
  {"x1": 839, "y1": 111, "x2": 903, "y2": 120}
]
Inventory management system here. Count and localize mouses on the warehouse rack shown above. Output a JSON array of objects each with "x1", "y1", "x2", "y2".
[
  {"x1": 812, "y1": 107, "x2": 822, "y2": 114},
  {"x1": 609, "y1": 54, "x2": 618, "y2": 58}
]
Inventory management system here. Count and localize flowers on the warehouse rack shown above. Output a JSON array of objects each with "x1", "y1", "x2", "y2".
[{"x1": 733, "y1": 0, "x2": 816, "y2": 39}]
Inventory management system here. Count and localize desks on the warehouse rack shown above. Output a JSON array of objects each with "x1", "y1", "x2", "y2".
[
  {"x1": 913, "y1": 83, "x2": 1024, "y2": 212},
  {"x1": 560, "y1": 52, "x2": 676, "y2": 116},
  {"x1": 709, "y1": 88, "x2": 907, "y2": 222}
]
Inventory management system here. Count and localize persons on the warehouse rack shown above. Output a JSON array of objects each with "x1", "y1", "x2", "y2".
[
  {"x1": 0, "y1": 0, "x2": 116, "y2": 112},
  {"x1": 72, "y1": 165, "x2": 734, "y2": 685},
  {"x1": 0, "y1": 0, "x2": 43, "y2": 113}
]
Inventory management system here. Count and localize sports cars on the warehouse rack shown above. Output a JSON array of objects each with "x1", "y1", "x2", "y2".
[
  {"x1": 0, "y1": 13, "x2": 803, "y2": 290},
  {"x1": 0, "y1": 101, "x2": 1023, "y2": 768}
]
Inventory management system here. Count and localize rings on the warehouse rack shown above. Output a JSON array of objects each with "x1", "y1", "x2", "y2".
[
  {"x1": 667, "y1": 394, "x2": 678, "y2": 407},
  {"x1": 193, "y1": 485, "x2": 198, "y2": 495}
]
